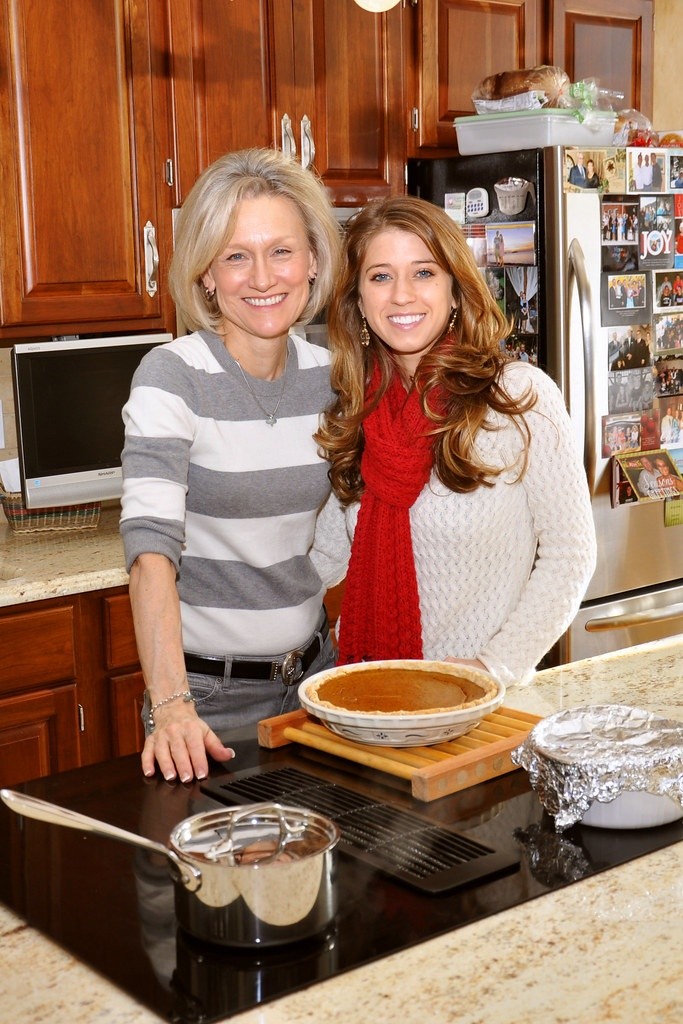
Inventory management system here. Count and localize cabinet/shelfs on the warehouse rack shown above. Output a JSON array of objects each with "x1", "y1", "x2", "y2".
[
  {"x1": 0, "y1": 2, "x2": 657, "y2": 353},
  {"x1": 0, "y1": 578, "x2": 152, "y2": 801}
]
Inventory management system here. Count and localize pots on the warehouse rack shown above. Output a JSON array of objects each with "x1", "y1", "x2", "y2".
[{"x1": 0, "y1": 789, "x2": 337, "y2": 949}]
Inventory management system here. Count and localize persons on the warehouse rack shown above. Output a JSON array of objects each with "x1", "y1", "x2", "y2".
[
  {"x1": 118, "y1": 147, "x2": 348, "y2": 781},
  {"x1": 567, "y1": 149, "x2": 683, "y2": 509},
  {"x1": 485, "y1": 227, "x2": 537, "y2": 366},
  {"x1": 308, "y1": 198, "x2": 597, "y2": 690}
]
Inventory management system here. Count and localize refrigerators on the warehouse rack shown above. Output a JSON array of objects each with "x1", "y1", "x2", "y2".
[{"x1": 409, "y1": 143, "x2": 683, "y2": 667}]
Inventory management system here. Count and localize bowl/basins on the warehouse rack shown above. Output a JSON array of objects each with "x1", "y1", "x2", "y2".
[
  {"x1": 535, "y1": 702, "x2": 683, "y2": 831},
  {"x1": 299, "y1": 660, "x2": 505, "y2": 749}
]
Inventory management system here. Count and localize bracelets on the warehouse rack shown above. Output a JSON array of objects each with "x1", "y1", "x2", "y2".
[{"x1": 149, "y1": 689, "x2": 196, "y2": 733}]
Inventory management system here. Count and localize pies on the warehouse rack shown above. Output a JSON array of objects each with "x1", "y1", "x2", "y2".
[{"x1": 308, "y1": 660, "x2": 499, "y2": 721}]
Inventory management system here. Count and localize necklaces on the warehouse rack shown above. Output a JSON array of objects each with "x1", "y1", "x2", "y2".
[{"x1": 232, "y1": 336, "x2": 288, "y2": 426}]
